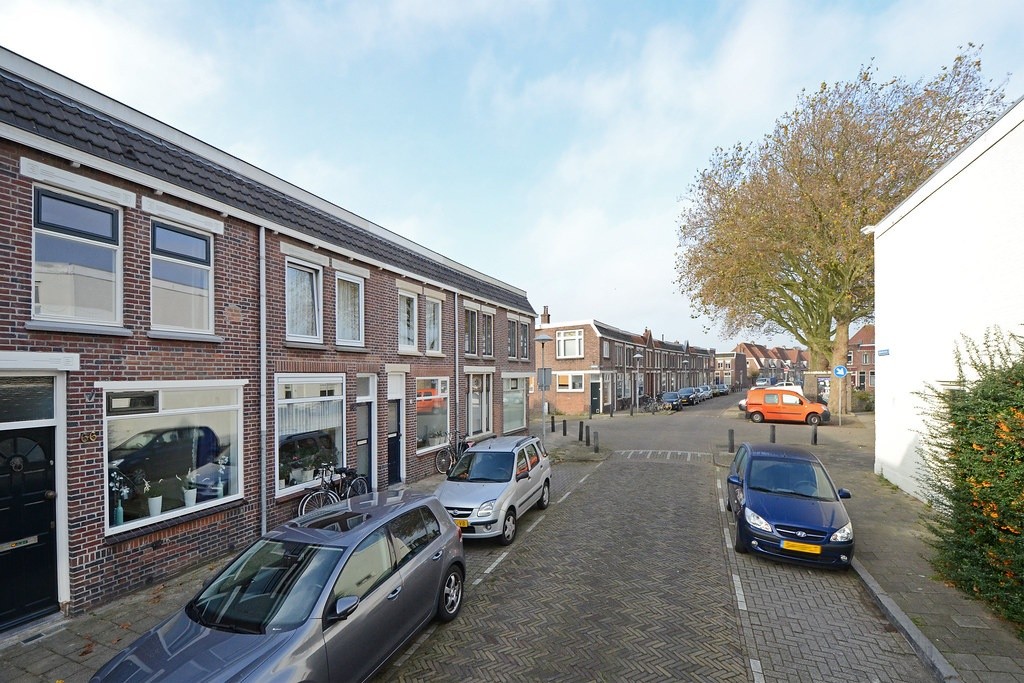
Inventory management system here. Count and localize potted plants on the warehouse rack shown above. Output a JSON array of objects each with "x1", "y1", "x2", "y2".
[{"x1": 278, "y1": 439, "x2": 337, "y2": 491}]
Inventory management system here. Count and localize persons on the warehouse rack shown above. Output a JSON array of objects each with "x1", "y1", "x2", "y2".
[{"x1": 732, "y1": 386, "x2": 735, "y2": 392}]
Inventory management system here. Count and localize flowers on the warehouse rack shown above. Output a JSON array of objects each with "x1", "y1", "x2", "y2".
[
  {"x1": 143, "y1": 478, "x2": 164, "y2": 498},
  {"x1": 174, "y1": 467, "x2": 211, "y2": 491},
  {"x1": 217, "y1": 455, "x2": 229, "y2": 478},
  {"x1": 109, "y1": 471, "x2": 130, "y2": 500}
]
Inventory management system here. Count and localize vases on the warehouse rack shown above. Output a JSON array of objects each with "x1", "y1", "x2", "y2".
[
  {"x1": 114, "y1": 499, "x2": 124, "y2": 523},
  {"x1": 217, "y1": 477, "x2": 225, "y2": 499},
  {"x1": 184, "y1": 489, "x2": 202, "y2": 510},
  {"x1": 149, "y1": 494, "x2": 162, "y2": 516}
]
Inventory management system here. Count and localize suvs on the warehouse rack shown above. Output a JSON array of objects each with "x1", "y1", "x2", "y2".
[
  {"x1": 431, "y1": 434, "x2": 553, "y2": 547},
  {"x1": 184, "y1": 428, "x2": 338, "y2": 502},
  {"x1": 83, "y1": 486, "x2": 468, "y2": 683}
]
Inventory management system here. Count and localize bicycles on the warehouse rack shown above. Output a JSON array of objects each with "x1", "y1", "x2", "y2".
[
  {"x1": 434, "y1": 430, "x2": 476, "y2": 475},
  {"x1": 297, "y1": 461, "x2": 370, "y2": 518}
]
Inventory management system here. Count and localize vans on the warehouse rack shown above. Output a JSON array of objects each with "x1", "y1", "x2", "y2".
[
  {"x1": 737, "y1": 384, "x2": 804, "y2": 411},
  {"x1": 745, "y1": 388, "x2": 831, "y2": 427}
]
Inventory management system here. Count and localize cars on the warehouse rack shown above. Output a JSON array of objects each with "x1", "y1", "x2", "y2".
[
  {"x1": 641, "y1": 382, "x2": 730, "y2": 415},
  {"x1": 108, "y1": 426, "x2": 220, "y2": 519},
  {"x1": 751, "y1": 378, "x2": 803, "y2": 390},
  {"x1": 725, "y1": 441, "x2": 855, "y2": 573}
]
